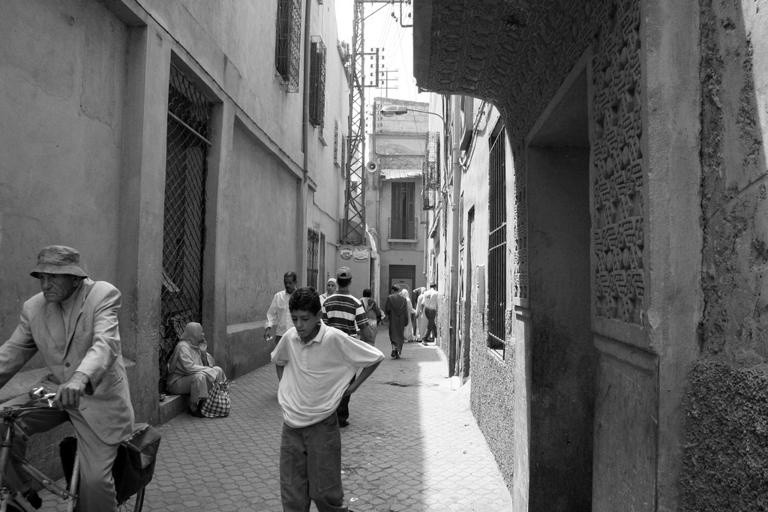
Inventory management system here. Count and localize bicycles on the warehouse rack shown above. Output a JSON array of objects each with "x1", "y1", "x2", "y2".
[{"x1": 1, "y1": 388, "x2": 146, "y2": 512}]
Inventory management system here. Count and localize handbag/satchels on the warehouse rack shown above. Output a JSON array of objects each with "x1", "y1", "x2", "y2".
[{"x1": 201, "y1": 376, "x2": 231, "y2": 419}]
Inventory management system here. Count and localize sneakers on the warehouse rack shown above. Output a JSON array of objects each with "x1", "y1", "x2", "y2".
[
  {"x1": 391, "y1": 349, "x2": 400, "y2": 359},
  {"x1": 6, "y1": 489, "x2": 43, "y2": 512}
]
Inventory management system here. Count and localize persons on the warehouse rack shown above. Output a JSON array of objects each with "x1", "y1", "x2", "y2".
[
  {"x1": 268, "y1": 285, "x2": 386, "y2": 511},
  {"x1": 383, "y1": 283, "x2": 409, "y2": 360},
  {"x1": 398, "y1": 282, "x2": 438, "y2": 347},
  {"x1": 319, "y1": 277, "x2": 337, "y2": 317},
  {"x1": 321, "y1": 266, "x2": 371, "y2": 428},
  {"x1": 0, "y1": 243, "x2": 135, "y2": 512},
  {"x1": 357, "y1": 288, "x2": 384, "y2": 347},
  {"x1": 165, "y1": 320, "x2": 230, "y2": 418},
  {"x1": 262, "y1": 270, "x2": 300, "y2": 383}
]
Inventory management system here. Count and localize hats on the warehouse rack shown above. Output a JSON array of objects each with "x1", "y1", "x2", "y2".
[
  {"x1": 337, "y1": 266, "x2": 352, "y2": 280},
  {"x1": 29, "y1": 245, "x2": 88, "y2": 281}
]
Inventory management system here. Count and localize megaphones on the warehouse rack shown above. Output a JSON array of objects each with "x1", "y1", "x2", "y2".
[{"x1": 366, "y1": 161, "x2": 377, "y2": 173}]
